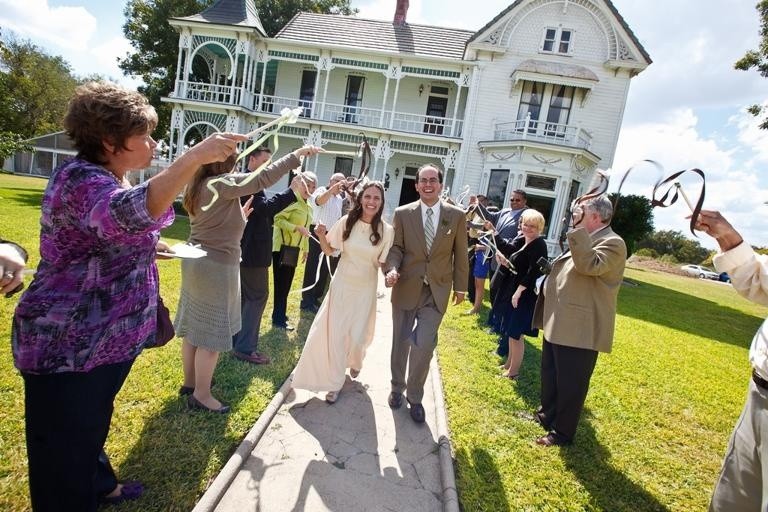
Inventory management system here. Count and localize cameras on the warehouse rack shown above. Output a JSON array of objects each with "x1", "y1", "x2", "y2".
[{"x1": 537, "y1": 256, "x2": 555, "y2": 276}]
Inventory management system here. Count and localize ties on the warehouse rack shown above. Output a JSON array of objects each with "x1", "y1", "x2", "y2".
[{"x1": 425, "y1": 208, "x2": 435, "y2": 254}]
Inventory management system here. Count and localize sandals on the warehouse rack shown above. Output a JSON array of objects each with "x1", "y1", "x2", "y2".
[
  {"x1": 350, "y1": 367, "x2": 360, "y2": 378},
  {"x1": 326, "y1": 390, "x2": 339, "y2": 403}
]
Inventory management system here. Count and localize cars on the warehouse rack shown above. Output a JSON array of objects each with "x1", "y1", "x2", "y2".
[
  {"x1": 718, "y1": 270, "x2": 732, "y2": 283},
  {"x1": 681, "y1": 264, "x2": 719, "y2": 281}
]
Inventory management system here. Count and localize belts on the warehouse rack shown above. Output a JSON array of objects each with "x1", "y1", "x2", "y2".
[{"x1": 753, "y1": 368, "x2": 768, "y2": 390}]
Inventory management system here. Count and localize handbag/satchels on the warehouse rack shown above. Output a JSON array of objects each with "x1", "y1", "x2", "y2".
[{"x1": 278, "y1": 245, "x2": 300, "y2": 271}]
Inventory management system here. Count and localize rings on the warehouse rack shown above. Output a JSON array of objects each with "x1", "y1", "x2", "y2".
[{"x1": 5, "y1": 270, "x2": 15, "y2": 280}]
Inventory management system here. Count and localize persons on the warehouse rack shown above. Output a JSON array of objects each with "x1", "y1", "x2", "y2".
[
  {"x1": 300, "y1": 173, "x2": 358, "y2": 316},
  {"x1": 465, "y1": 194, "x2": 500, "y2": 314},
  {"x1": 232, "y1": 145, "x2": 302, "y2": 365},
  {"x1": 532, "y1": 192, "x2": 627, "y2": 446},
  {"x1": 380, "y1": 162, "x2": 471, "y2": 422},
  {"x1": 289, "y1": 180, "x2": 401, "y2": 404},
  {"x1": 683, "y1": 207, "x2": 768, "y2": 512},
  {"x1": 0, "y1": 236, "x2": 29, "y2": 299},
  {"x1": 273, "y1": 171, "x2": 319, "y2": 332},
  {"x1": 11, "y1": 86, "x2": 250, "y2": 512},
  {"x1": 174, "y1": 144, "x2": 326, "y2": 415},
  {"x1": 484, "y1": 209, "x2": 548, "y2": 383},
  {"x1": 471, "y1": 190, "x2": 529, "y2": 335}
]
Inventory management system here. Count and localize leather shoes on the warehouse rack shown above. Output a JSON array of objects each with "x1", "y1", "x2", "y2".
[
  {"x1": 237, "y1": 351, "x2": 267, "y2": 363},
  {"x1": 536, "y1": 434, "x2": 570, "y2": 444},
  {"x1": 388, "y1": 392, "x2": 404, "y2": 408},
  {"x1": 274, "y1": 322, "x2": 294, "y2": 331},
  {"x1": 101, "y1": 483, "x2": 143, "y2": 503},
  {"x1": 406, "y1": 397, "x2": 425, "y2": 422}
]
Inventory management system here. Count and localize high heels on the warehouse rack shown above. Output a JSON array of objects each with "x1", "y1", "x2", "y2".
[
  {"x1": 179, "y1": 376, "x2": 217, "y2": 394},
  {"x1": 188, "y1": 395, "x2": 230, "y2": 413}
]
